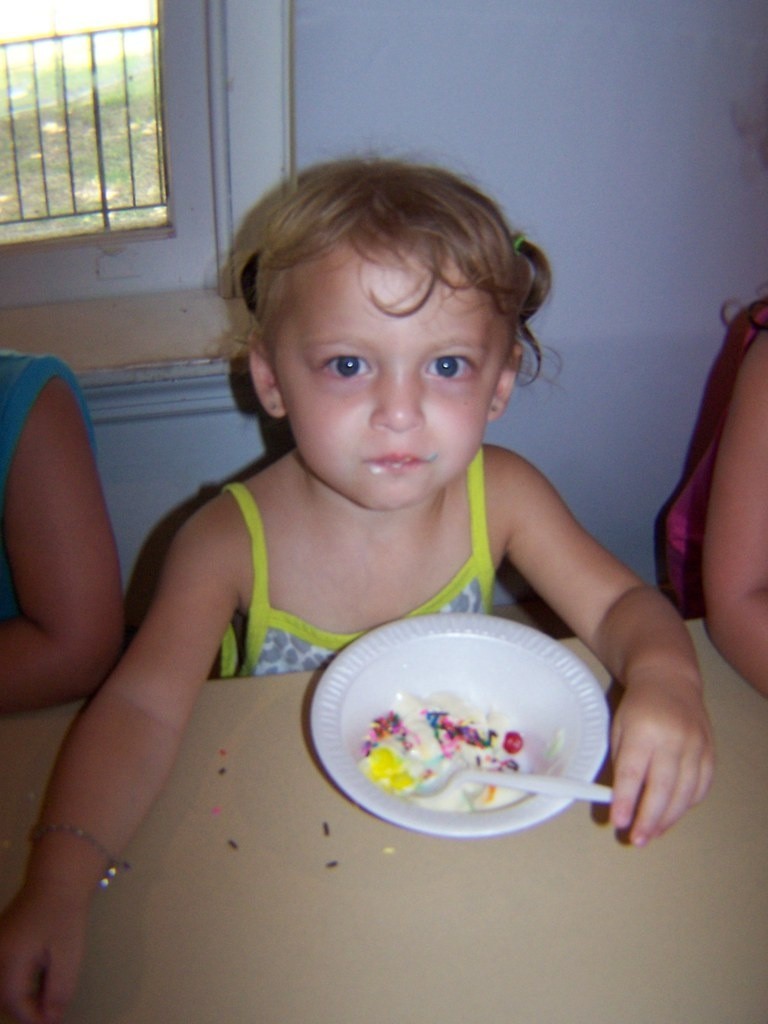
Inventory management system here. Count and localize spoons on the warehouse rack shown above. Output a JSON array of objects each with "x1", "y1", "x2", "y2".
[{"x1": 407, "y1": 760, "x2": 612, "y2": 802}]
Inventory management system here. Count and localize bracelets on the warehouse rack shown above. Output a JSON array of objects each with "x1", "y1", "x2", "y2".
[{"x1": 31, "y1": 824, "x2": 114, "y2": 863}]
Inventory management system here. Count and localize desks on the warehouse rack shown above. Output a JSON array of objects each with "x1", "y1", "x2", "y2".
[{"x1": 0, "y1": 622, "x2": 768, "y2": 1024}]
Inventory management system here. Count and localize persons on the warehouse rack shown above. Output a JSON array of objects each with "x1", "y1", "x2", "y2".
[
  {"x1": 0, "y1": 349, "x2": 127, "y2": 716},
  {"x1": 0, "y1": 160, "x2": 716, "y2": 1024},
  {"x1": 663, "y1": 297, "x2": 768, "y2": 702}
]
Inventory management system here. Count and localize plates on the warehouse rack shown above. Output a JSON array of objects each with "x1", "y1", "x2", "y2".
[{"x1": 310, "y1": 612, "x2": 609, "y2": 835}]
